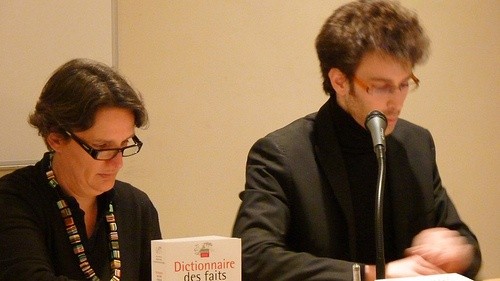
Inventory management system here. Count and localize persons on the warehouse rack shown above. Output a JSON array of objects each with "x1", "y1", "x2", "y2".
[
  {"x1": 0, "y1": 57, "x2": 163, "y2": 281},
  {"x1": 233, "y1": 0, "x2": 483, "y2": 281}
]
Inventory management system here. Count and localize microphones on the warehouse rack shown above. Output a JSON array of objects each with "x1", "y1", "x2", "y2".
[{"x1": 365, "y1": 109, "x2": 388, "y2": 281}]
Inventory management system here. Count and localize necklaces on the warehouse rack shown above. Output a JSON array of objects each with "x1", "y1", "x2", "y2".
[{"x1": 46, "y1": 168, "x2": 121, "y2": 281}]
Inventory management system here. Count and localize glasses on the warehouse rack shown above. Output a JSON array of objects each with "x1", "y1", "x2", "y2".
[
  {"x1": 352, "y1": 69, "x2": 420, "y2": 99},
  {"x1": 65, "y1": 127, "x2": 143, "y2": 160}
]
